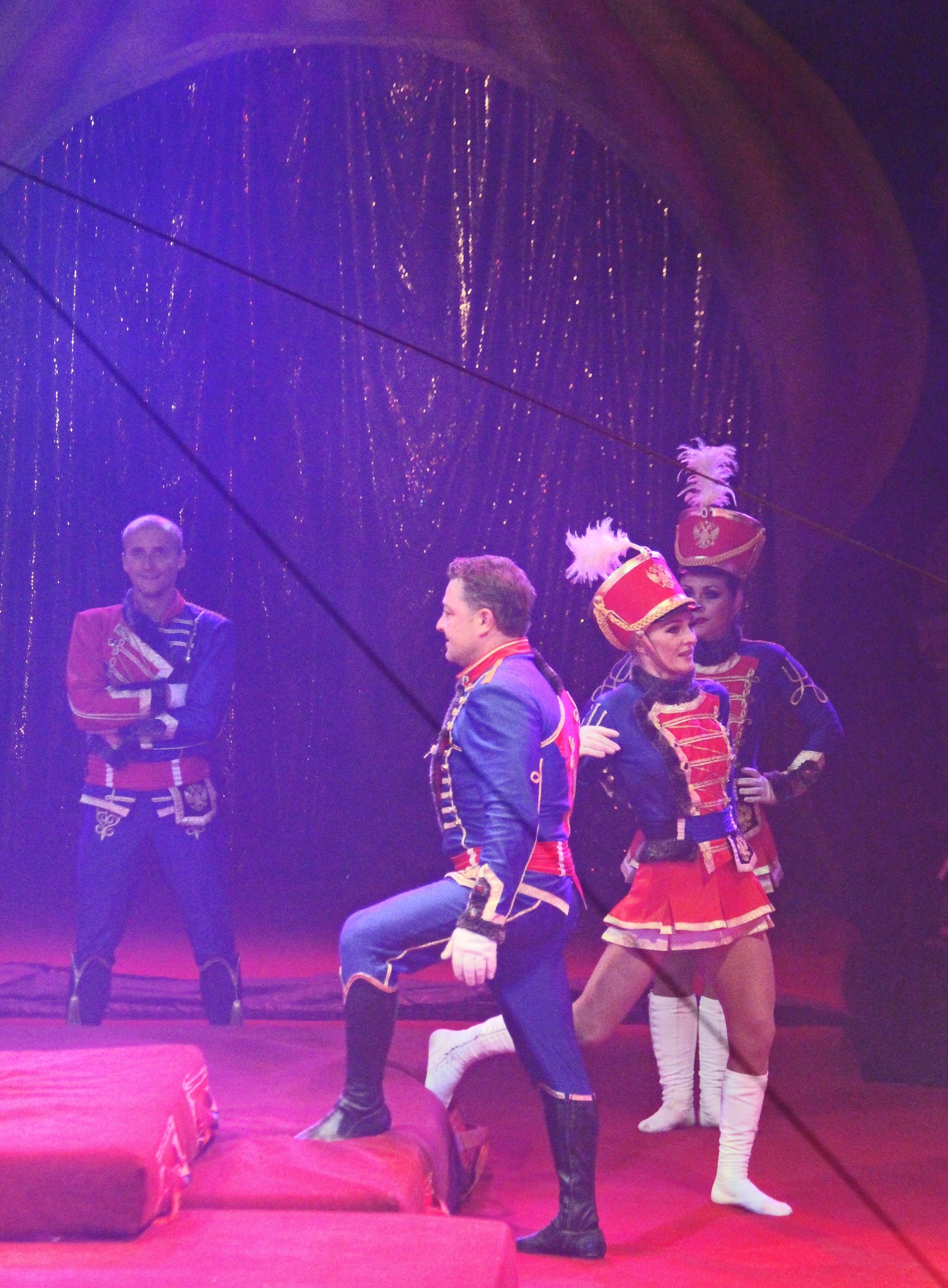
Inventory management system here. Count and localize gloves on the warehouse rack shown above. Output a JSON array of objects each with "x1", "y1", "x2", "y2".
[
  {"x1": 735, "y1": 768, "x2": 779, "y2": 806},
  {"x1": 166, "y1": 680, "x2": 188, "y2": 708},
  {"x1": 575, "y1": 726, "x2": 621, "y2": 758},
  {"x1": 439, "y1": 930, "x2": 499, "y2": 987},
  {"x1": 101, "y1": 730, "x2": 122, "y2": 750}
]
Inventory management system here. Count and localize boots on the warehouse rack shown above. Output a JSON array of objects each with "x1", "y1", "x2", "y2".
[
  {"x1": 638, "y1": 989, "x2": 698, "y2": 1134},
  {"x1": 697, "y1": 996, "x2": 730, "y2": 1129},
  {"x1": 711, "y1": 1069, "x2": 793, "y2": 1217},
  {"x1": 424, "y1": 1013, "x2": 516, "y2": 1112},
  {"x1": 197, "y1": 953, "x2": 244, "y2": 1027},
  {"x1": 292, "y1": 963, "x2": 399, "y2": 1142},
  {"x1": 515, "y1": 1083, "x2": 606, "y2": 1259},
  {"x1": 64, "y1": 950, "x2": 112, "y2": 1027}
]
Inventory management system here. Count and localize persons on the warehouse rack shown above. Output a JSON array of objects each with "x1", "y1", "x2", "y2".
[
  {"x1": 282, "y1": 556, "x2": 607, "y2": 1261},
  {"x1": 425, "y1": 516, "x2": 797, "y2": 1217},
  {"x1": 580, "y1": 435, "x2": 846, "y2": 1130},
  {"x1": 66, "y1": 516, "x2": 245, "y2": 1030}
]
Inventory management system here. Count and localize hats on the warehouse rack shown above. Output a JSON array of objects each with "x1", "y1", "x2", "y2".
[
  {"x1": 563, "y1": 515, "x2": 700, "y2": 676},
  {"x1": 674, "y1": 437, "x2": 767, "y2": 584}
]
